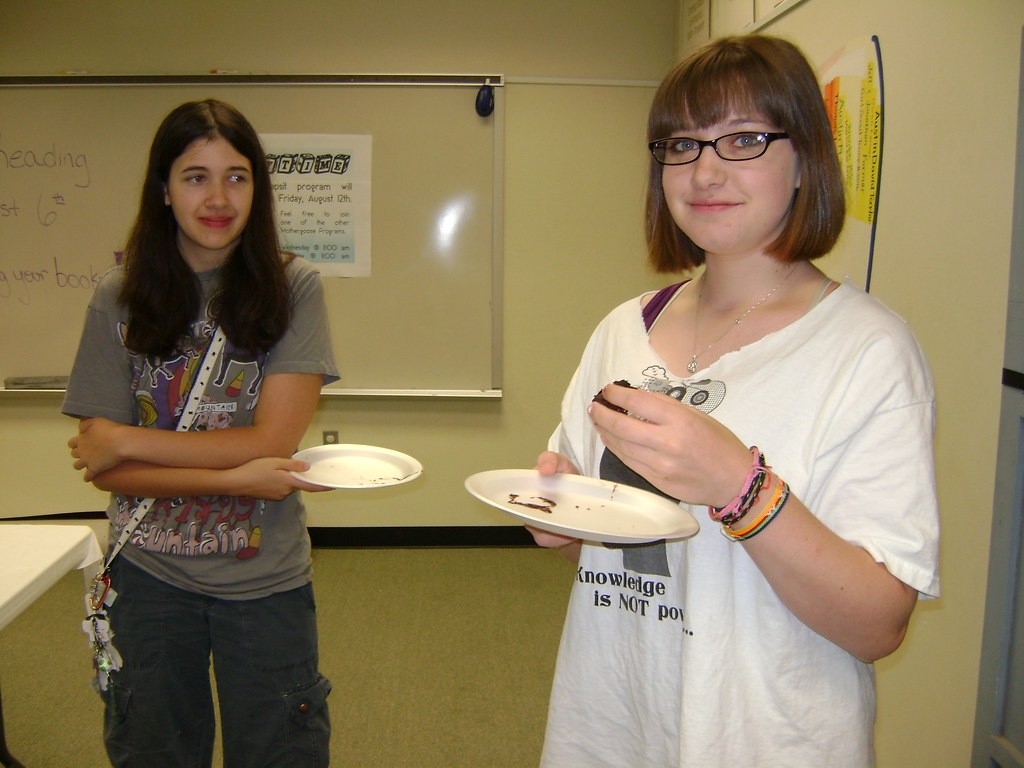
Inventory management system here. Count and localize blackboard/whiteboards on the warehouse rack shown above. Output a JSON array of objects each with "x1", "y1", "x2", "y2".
[{"x1": 0, "y1": 75, "x2": 507, "y2": 399}]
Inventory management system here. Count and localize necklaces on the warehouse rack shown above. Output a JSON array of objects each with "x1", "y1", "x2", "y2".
[{"x1": 688, "y1": 263, "x2": 799, "y2": 373}]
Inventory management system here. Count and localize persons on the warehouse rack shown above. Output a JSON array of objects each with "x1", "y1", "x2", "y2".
[
  {"x1": 520, "y1": 35, "x2": 941, "y2": 768},
  {"x1": 61, "y1": 98, "x2": 341, "y2": 768}
]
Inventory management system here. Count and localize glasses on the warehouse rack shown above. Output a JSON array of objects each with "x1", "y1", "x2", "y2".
[{"x1": 648, "y1": 132, "x2": 790, "y2": 165}]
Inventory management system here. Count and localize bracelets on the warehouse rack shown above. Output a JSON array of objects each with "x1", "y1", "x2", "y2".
[{"x1": 708, "y1": 446, "x2": 789, "y2": 541}]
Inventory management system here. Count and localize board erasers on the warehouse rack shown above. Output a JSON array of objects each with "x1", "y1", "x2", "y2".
[{"x1": 4, "y1": 376, "x2": 70, "y2": 390}]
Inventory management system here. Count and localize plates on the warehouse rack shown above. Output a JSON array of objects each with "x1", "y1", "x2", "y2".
[
  {"x1": 288, "y1": 444, "x2": 423, "y2": 488},
  {"x1": 464, "y1": 469, "x2": 700, "y2": 544}
]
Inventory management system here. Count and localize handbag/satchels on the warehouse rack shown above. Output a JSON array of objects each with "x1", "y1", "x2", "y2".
[{"x1": 83, "y1": 579, "x2": 123, "y2": 692}]
